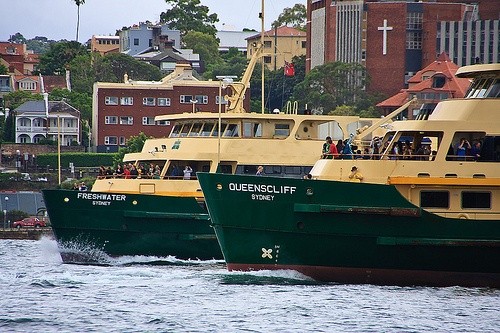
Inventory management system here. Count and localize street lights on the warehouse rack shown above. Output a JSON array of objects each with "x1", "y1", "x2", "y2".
[
  {"x1": 15, "y1": 149, "x2": 20, "y2": 182},
  {"x1": 4, "y1": 196, "x2": 8, "y2": 228},
  {"x1": 23, "y1": 152, "x2": 29, "y2": 173}
]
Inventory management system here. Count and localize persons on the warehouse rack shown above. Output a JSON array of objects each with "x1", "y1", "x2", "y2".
[
  {"x1": 95, "y1": 160, "x2": 194, "y2": 179},
  {"x1": 255, "y1": 166, "x2": 264, "y2": 176},
  {"x1": 70, "y1": 182, "x2": 87, "y2": 191},
  {"x1": 322, "y1": 136, "x2": 484, "y2": 161}
]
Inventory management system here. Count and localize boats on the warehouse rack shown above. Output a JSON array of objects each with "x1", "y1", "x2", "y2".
[
  {"x1": 39, "y1": 42, "x2": 439, "y2": 267},
  {"x1": 196, "y1": 56, "x2": 500, "y2": 281}
]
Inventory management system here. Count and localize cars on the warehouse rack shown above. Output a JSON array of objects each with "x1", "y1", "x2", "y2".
[{"x1": 12, "y1": 217, "x2": 47, "y2": 229}]
